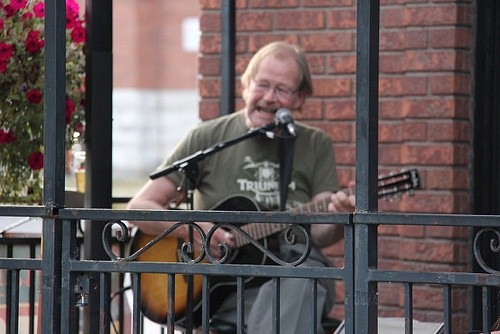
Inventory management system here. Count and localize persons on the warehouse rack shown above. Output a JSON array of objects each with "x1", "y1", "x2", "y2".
[{"x1": 127, "y1": 41, "x2": 356, "y2": 334}]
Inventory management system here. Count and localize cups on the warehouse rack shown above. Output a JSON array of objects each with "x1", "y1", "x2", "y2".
[{"x1": 73, "y1": 151, "x2": 85, "y2": 192}]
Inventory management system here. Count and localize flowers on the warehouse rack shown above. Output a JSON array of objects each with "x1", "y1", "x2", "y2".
[{"x1": 0, "y1": 0, "x2": 87, "y2": 205}]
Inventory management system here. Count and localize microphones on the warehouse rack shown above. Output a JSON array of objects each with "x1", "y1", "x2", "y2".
[{"x1": 275, "y1": 107, "x2": 298, "y2": 137}]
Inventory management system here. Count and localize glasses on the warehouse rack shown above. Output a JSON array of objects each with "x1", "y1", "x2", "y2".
[{"x1": 250, "y1": 79, "x2": 301, "y2": 100}]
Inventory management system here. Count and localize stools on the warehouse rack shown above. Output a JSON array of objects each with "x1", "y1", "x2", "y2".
[{"x1": 198, "y1": 317, "x2": 342, "y2": 334}]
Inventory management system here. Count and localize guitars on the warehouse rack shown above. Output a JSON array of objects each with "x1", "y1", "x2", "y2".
[{"x1": 130, "y1": 168, "x2": 422, "y2": 331}]
